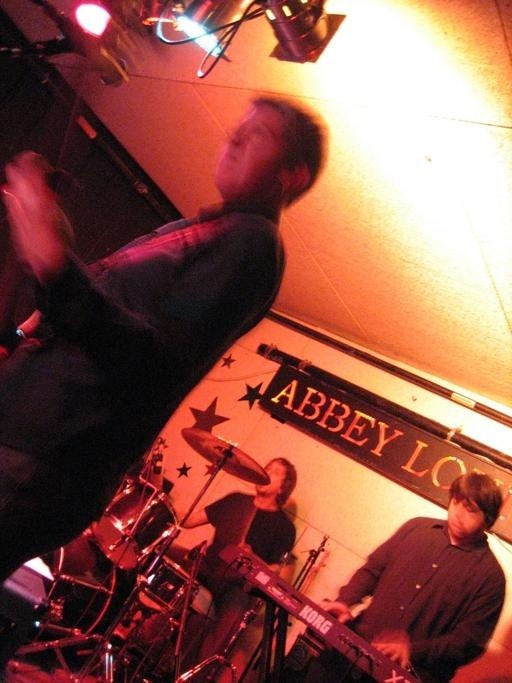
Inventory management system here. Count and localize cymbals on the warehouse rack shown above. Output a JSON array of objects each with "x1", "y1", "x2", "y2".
[{"x1": 182, "y1": 428, "x2": 270, "y2": 484}]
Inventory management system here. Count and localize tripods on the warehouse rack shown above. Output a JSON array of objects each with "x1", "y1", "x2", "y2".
[
  {"x1": 17, "y1": 457, "x2": 234, "y2": 683},
  {"x1": 173, "y1": 598, "x2": 264, "y2": 682}
]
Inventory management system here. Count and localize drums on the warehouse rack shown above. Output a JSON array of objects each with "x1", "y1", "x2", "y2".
[
  {"x1": 22, "y1": 529, "x2": 117, "y2": 664},
  {"x1": 137, "y1": 550, "x2": 202, "y2": 620},
  {"x1": 92, "y1": 479, "x2": 180, "y2": 570}
]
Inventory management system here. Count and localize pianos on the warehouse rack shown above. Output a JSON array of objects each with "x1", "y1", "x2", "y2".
[{"x1": 218, "y1": 542, "x2": 422, "y2": 683}]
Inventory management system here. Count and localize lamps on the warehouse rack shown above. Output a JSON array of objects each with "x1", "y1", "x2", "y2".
[
  {"x1": 261, "y1": 0, "x2": 347, "y2": 65},
  {"x1": 176, "y1": 1, "x2": 256, "y2": 63}
]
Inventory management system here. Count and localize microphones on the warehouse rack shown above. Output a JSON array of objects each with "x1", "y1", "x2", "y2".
[{"x1": 52, "y1": 9, "x2": 128, "y2": 89}]
